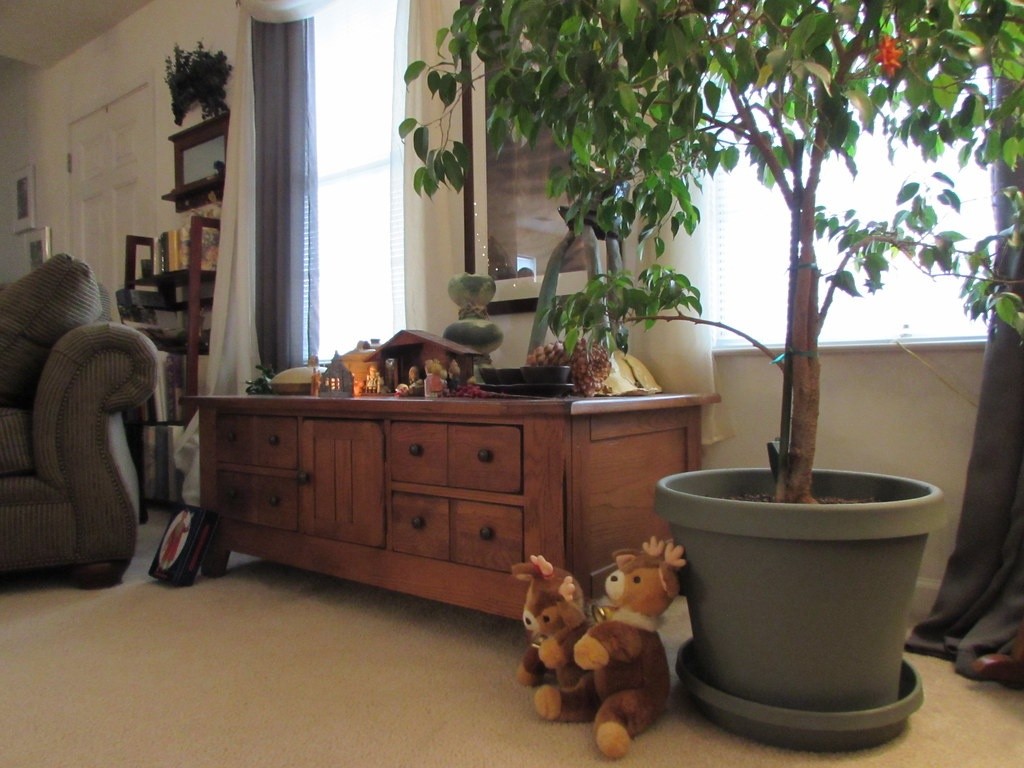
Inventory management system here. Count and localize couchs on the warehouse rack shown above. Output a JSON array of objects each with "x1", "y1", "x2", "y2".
[{"x1": 0, "y1": 320, "x2": 161, "y2": 591}]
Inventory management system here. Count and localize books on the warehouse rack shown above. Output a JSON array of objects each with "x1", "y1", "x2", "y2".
[
  {"x1": 140, "y1": 361, "x2": 185, "y2": 420},
  {"x1": 152, "y1": 222, "x2": 218, "y2": 274},
  {"x1": 143, "y1": 425, "x2": 187, "y2": 504}
]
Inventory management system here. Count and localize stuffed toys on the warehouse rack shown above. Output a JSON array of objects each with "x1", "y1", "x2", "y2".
[{"x1": 512, "y1": 535, "x2": 687, "y2": 761}]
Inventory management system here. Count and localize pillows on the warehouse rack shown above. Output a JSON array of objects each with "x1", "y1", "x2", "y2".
[{"x1": 0, "y1": 252, "x2": 102, "y2": 404}]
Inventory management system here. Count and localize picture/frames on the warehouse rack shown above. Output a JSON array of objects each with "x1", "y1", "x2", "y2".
[
  {"x1": 460, "y1": 0, "x2": 623, "y2": 319},
  {"x1": 26, "y1": 226, "x2": 51, "y2": 274},
  {"x1": 15, "y1": 162, "x2": 37, "y2": 234}
]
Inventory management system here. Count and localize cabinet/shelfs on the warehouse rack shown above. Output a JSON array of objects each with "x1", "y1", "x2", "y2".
[
  {"x1": 121, "y1": 216, "x2": 221, "y2": 521},
  {"x1": 175, "y1": 391, "x2": 720, "y2": 623}
]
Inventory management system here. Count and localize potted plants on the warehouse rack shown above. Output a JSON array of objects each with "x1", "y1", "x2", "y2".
[{"x1": 396, "y1": 0, "x2": 1024, "y2": 751}]
